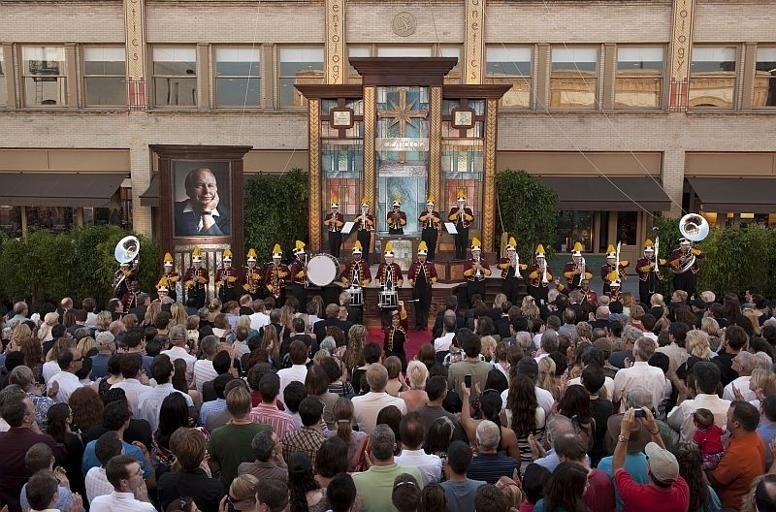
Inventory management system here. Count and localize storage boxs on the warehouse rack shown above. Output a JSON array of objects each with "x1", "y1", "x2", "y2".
[{"x1": 431, "y1": 258, "x2": 466, "y2": 284}]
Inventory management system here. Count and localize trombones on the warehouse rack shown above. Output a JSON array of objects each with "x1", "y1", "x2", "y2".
[{"x1": 646, "y1": 234, "x2": 661, "y2": 295}]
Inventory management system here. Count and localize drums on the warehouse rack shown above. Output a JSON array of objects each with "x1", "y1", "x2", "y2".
[
  {"x1": 346, "y1": 283, "x2": 366, "y2": 307},
  {"x1": 306, "y1": 254, "x2": 339, "y2": 288},
  {"x1": 377, "y1": 289, "x2": 400, "y2": 310}
]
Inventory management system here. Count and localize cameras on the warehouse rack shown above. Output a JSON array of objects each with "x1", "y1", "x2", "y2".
[{"x1": 634, "y1": 409, "x2": 646, "y2": 418}]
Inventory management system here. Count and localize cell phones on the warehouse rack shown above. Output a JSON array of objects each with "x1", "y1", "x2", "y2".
[{"x1": 464, "y1": 374, "x2": 472, "y2": 389}]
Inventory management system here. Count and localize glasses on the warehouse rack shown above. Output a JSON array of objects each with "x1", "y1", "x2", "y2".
[
  {"x1": 74, "y1": 356, "x2": 83, "y2": 361},
  {"x1": 70, "y1": 410, "x2": 75, "y2": 416},
  {"x1": 228, "y1": 494, "x2": 246, "y2": 504},
  {"x1": 56, "y1": 477, "x2": 61, "y2": 488}
]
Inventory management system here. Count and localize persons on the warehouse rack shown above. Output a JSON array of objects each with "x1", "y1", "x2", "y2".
[
  {"x1": 497, "y1": 236, "x2": 529, "y2": 305},
  {"x1": 112, "y1": 263, "x2": 138, "y2": 300},
  {"x1": 668, "y1": 237, "x2": 705, "y2": 300},
  {"x1": 174, "y1": 168, "x2": 230, "y2": 236},
  {"x1": 323, "y1": 195, "x2": 345, "y2": 261},
  {"x1": 407, "y1": 240, "x2": 438, "y2": 331},
  {"x1": 387, "y1": 193, "x2": 407, "y2": 235},
  {"x1": 182, "y1": 245, "x2": 209, "y2": 310},
  {"x1": 353, "y1": 195, "x2": 374, "y2": 264},
  {"x1": 635, "y1": 238, "x2": 668, "y2": 308},
  {"x1": 418, "y1": 193, "x2": 441, "y2": 260},
  {"x1": 287, "y1": 239, "x2": 309, "y2": 314},
  {"x1": 241, "y1": 247, "x2": 264, "y2": 300},
  {"x1": 214, "y1": 247, "x2": 239, "y2": 304},
  {"x1": 524, "y1": 242, "x2": 553, "y2": 308},
  {"x1": 374, "y1": 239, "x2": 404, "y2": 332},
  {"x1": 600, "y1": 243, "x2": 630, "y2": 295},
  {"x1": 339, "y1": 239, "x2": 372, "y2": 324},
  {"x1": 186, "y1": 297, "x2": 199, "y2": 317},
  {"x1": 563, "y1": 241, "x2": 593, "y2": 290},
  {"x1": 463, "y1": 236, "x2": 492, "y2": 309},
  {"x1": 448, "y1": 190, "x2": 474, "y2": 260},
  {"x1": 264, "y1": 242, "x2": 291, "y2": 308},
  {"x1": 154, "y1": 252, "x2": 182, "y2": 302},
  {"x1": 578, "y1": 279, "x2": 598, "y2": 312}
]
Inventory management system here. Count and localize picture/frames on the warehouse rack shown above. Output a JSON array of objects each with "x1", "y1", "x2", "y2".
[{"x1": 172, "y1": 158, "x2": 233, "y2": 242}]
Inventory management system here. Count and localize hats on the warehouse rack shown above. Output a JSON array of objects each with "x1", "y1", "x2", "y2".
[
  {"x1": 457, "y1": 191, "x2": 466, "y2": 202},
  {"x1": 417, "y1": 241, "x2": 427, "y2": 256},
  {"x1": 119, "y1": 261, "x2": 132, "y2": 266},
  {"x1": 246, "y1": 248, "x2": 257, "y2": 262},
  {"x1": 535, "y1": 244, "x2": 545, "y2": 258},
  {"x1": 679, "y1": 238, "x2": 691, "y2": 245},
  {"x1": 292, "y1": 240, "x2": 305, "y2": 255},
  {"x1": 605, "y1": 245, "x2": 616, "y2": 259},
  {"x1": 645, "y1": 441, "x2": 679, "y2": 484},
  {"x1": 163, "y1": 252, "x2": 173, "y2": 267},
  {"x1": 192, "y1": 246, "x2": 202, "y2": 263},
  {"x1": 427, "y1": 194, "x2": 435, "y2": 206},
  {"x1": 331, "y1": 199, "x2": 338, "y2": 209},
  {"x1": 505, "y1": 237, "x2": 517, "y2": 251},
  {"x1": 222, "y1": 248, "x2": 233, "y2": 262},
  {"x1": 383, "y1": 241, "x2": 394, "y2": 258},
  {"x1": 361, "y1": 198, "x2": 370, "y2": 208},
  {"x1": 352, "y1": 241, "x2": 362, "y2": 255},
  {"x1": 470, "y1": 237, "x2": 481, "y2": 251},
  {"x1": 624, "y1": 325, "x2": 644, "y2": 344},
  {"x1": 95, "y1": 332, "x2": 115, "y2": 343},
  {"x1": 392, "y1": 196, "x2": 401, "y2": 207},
  {"x1": 643, "y1": 240, "x2": 654, "y2": 253},
  {"x1": 157, "y1": 277, "x2": 169, "y2": 292},
  {"x1": 272, "y1": 243, "x2": 282, "y2": 259},
  {"x1": 571, "y1": 242, "x2": 582, "y2": 258}
]
